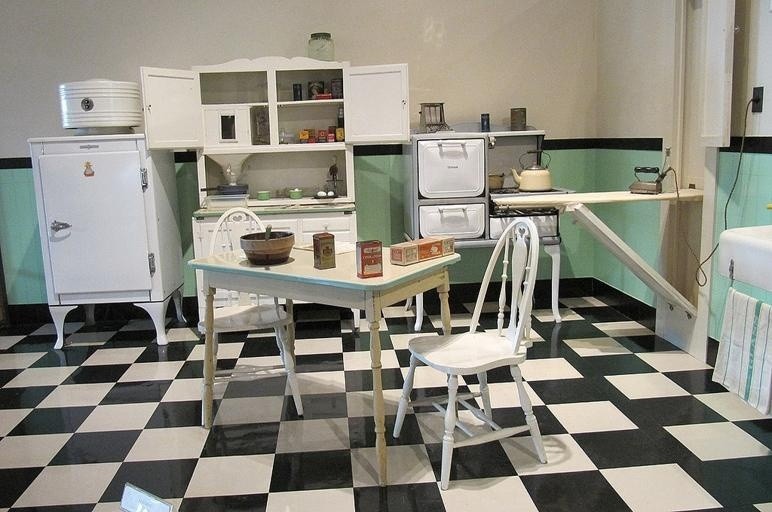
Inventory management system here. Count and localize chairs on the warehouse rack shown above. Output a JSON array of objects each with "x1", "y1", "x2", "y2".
[
  {"x1": 390, "y1": 215, "x2": 549, "y2": 491},
  {"x1": 196, "y1": 206, "x2": 306, "y2": 426}
]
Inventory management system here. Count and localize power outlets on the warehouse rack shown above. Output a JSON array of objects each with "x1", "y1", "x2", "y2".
[{"x1": 752, "y1": 86, "x2": 763, "y2": 112}]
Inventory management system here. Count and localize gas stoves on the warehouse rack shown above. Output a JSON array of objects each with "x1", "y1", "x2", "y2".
[{"x1": 491, "y1": 187, "x2": 568, "y2": 197}]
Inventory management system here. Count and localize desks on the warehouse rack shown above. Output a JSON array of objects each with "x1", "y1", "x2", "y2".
[{"x1": 187, "y1": 241, "x2": 462, "y2": 493}]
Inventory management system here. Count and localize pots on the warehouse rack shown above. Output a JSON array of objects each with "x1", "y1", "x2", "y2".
[
  {"x1": 200, "y1": 183, "x2": 248, "y2": 195},
  {"x1": 489, "y1": 173, "x2": 504, "y2": 190}
]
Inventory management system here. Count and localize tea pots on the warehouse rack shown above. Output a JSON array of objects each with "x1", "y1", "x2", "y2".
[{"x1": 510, "y1": 150, "x2": 551, "y2": 190}]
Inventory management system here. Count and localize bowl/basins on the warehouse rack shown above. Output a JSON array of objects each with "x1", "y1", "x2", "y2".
[{"x1": 240, "y1": 231, "x2": 294, "y2": 265}]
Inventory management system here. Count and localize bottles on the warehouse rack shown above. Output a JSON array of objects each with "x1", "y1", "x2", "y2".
[
  {"x1": 307, "y1": 32, "x2": 335, "y2": 59},
  {"x1": 661, "y1": 147, "x2": 675, "y2": 193},
  {"x1": 279, "y1": 128, "x2": 288, "y2": 144}
]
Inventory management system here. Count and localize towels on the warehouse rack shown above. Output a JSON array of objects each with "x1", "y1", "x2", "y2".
[{"x1": 710, "y1": 287, "x2": 772, "y2": 417}]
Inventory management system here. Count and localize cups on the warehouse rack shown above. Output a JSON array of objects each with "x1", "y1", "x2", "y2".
[
  {"x1": 510, "y1": 107, "x2": 527, "y2": 130},
  {"x1": 481, "y1": 113, "x2": 490, "y2": 131},
  {"x1": 289, "y1": 190, "x2": 302, "y2": 199},
  {"x1": 293, "y1": 83, "x2": 302, "y2": 101},
  {"x1": 257, "y1": 191, "x2": 270, "y2": 200}
]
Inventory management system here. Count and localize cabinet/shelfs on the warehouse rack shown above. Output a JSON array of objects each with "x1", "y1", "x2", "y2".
[
  {"x1": 190, "y1": 148, "x2": 362, "y2": 333},
  {"x1": 26, "y1": 134, "x2": 190, "y2": 351},
  {"x1": 139, "y1": 55, "x2": 412, "y2": 149}
]
